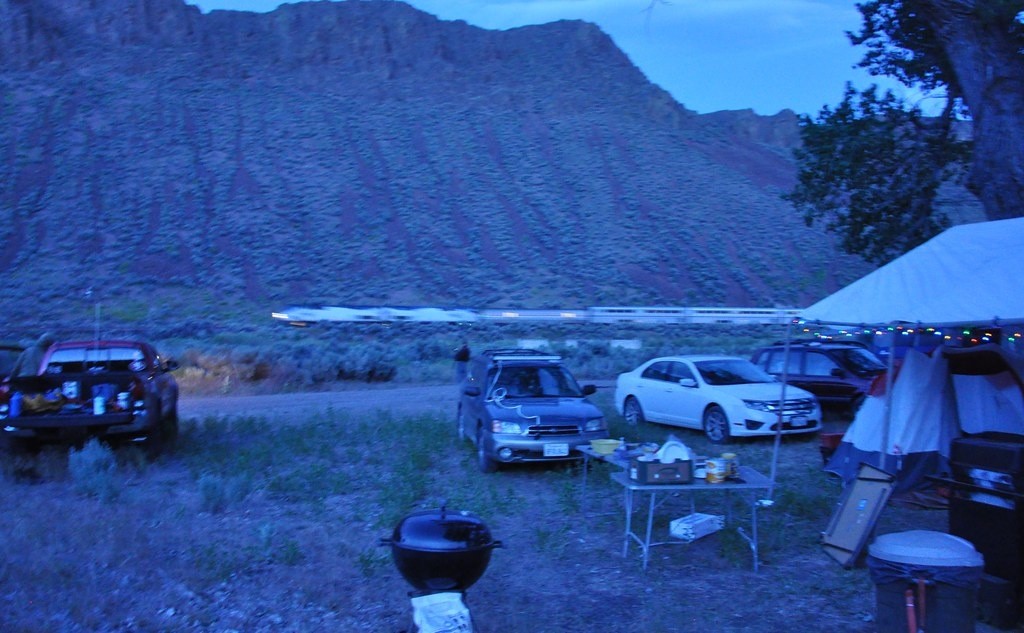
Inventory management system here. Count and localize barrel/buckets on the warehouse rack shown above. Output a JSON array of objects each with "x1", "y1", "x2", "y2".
[
  {"x1": 705, "y1": 460, "x2": 729, "y2": 484},
  {"x1": 718, "y1": 458, "x2": 739, "y2": 481}
]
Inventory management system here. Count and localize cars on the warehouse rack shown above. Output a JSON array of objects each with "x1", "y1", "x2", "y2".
[
  {"x1": 750, "y1": 340, "x2": 888, "y2": 417},
  {"x1": 613, "y1": 356, "x2": 822, "y2": 443}
]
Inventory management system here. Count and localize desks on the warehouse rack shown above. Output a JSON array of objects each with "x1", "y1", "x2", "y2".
[
  {"x1": 609, "y1": 467, "x2": 779, "y2": 574},
  {"x1": 811, "y1": 461, "x2": 901, "y2": 568},
  {"x1": 575, "y1": 443, "x2": 712, "y2": 550}
]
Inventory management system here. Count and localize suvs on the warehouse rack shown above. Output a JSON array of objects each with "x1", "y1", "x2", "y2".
[
  {"x1": 456, "y1": 349, "x2": 612, "y2": 472},
  {"x1": 0, "y1": 340, "x2": 179, "y2": 446}
]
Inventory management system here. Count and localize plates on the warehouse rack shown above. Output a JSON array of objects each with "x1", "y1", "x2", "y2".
[{"x1": 660, "y1": 441, "x2": 689, "y2": 463}]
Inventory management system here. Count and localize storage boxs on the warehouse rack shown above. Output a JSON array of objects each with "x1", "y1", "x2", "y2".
[{"x1": 818, "y1": 433, "x2": 844, "y2": 478}]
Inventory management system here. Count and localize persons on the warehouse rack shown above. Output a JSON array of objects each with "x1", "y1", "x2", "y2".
[{"x1": 12, "y1": 332, "x2": 56, "y2": 377}]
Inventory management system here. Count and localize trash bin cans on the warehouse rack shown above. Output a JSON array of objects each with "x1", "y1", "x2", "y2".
[{"x1": 864, "y1": 528, "x2": 984, "y2": 633}]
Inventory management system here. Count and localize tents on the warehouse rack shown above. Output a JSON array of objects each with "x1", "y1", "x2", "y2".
[
  {"x1": 766, "y1": 216, "x2": 1024, "y2": 503},
  {"x1": 822, "y1": 350, "x2": 1024, "y2": 491}
]
